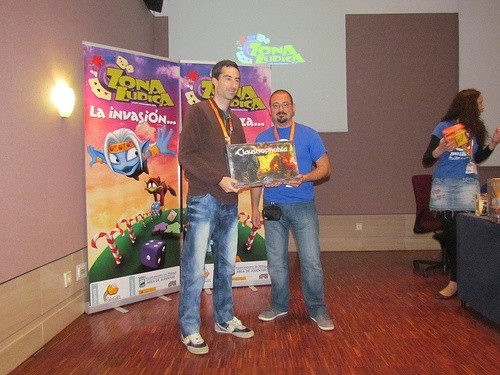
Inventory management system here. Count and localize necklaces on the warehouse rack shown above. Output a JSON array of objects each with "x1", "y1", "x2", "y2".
[
  {"x1": 274, "y1": 120, "x2": 295, "y2": 141},
  {"x1": 208, "y1": 99, "x2": 233, "y2": 144}
]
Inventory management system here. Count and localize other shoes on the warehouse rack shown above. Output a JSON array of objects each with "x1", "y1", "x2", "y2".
[{"x1": 435, "y1": 290, "x2": 457, "y2": 299}]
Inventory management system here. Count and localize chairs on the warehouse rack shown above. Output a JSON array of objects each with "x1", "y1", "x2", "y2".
[{"x1": 411, "y1": 174, "x2": 454, "y2": 274}]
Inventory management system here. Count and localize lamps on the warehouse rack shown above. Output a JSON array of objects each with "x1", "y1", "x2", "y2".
[{"x1": 57, "y1": 88, "x2": 75, "y2": 118}]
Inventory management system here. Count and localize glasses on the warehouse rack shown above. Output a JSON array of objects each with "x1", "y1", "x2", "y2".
[{"x1": 271, "y1": 102, "x2": 291, "y2": 108}]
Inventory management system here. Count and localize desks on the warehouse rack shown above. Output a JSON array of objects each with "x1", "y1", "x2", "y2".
[{"x1": 456, "y1": 211, "x2": 500, "y2": 327}]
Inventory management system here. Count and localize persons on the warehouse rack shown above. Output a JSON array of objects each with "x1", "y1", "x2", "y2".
[
  {"x1": 178, "y1": 60, "x2": 254, "y2": 355},
  {"x1": 250, "y1": 89, "x2": 334, "y2": 330},
  {"x1": 422, "y1": 88, "x2": 500, "y2": 299}
]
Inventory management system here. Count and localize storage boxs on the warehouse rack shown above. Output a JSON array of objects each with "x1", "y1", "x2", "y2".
[{"x1": 224, "y1": 140, "x2": 299, "y2": 188}]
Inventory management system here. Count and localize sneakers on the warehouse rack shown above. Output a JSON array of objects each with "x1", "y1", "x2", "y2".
[
  {"x1": 180, "y1": 332, "x2": 209, "y2": 354},
  {"x1": 214, "y1": 317, "x2": 254, "y2": 339},
  {"x1": 258, "y1": 308, "x2": 289, "y2": 320},
  {"x1": 310, "y1": 314, "x2": 334, "y2": 331}
]
